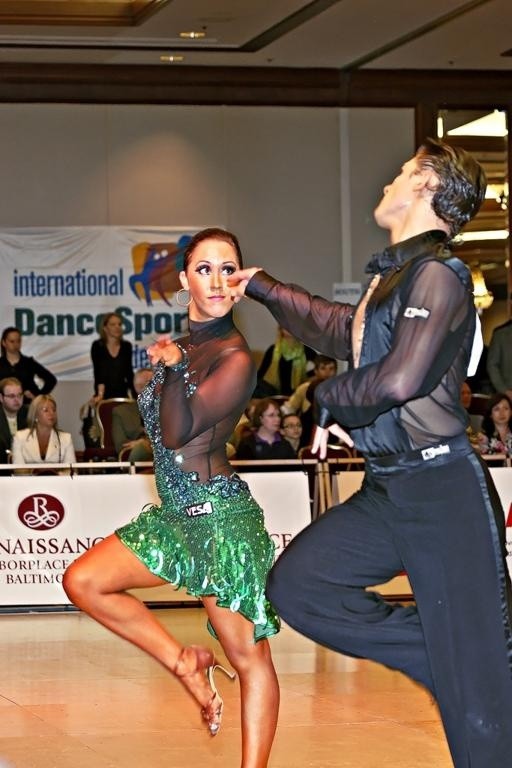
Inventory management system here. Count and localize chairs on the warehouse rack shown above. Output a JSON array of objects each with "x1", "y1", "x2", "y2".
[
  {"x1": 246, "y1": 394, "x2": 317, "y2": 472},
  {"x1": 95, "y1": 398, "x2": 154, "y2": 474},
  {"x1": 466, "y1": 394, "x2": 508, "y2": 467}
]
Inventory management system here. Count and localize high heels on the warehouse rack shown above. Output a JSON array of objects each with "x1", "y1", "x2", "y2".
[{"x1": 174, "y1": 644, "x2": 236, "y2": 737}]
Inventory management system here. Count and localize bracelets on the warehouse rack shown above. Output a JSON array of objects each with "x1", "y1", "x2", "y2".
[
  {"x1": 168, "y1": 341, "x2": 192, "y2": 372},
  {"x1": 98, "y1": 391, "x2": 105, "y2": 398}
]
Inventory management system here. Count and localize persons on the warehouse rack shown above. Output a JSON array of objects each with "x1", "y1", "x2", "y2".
[
  {"x1": 87, "y1": 310, "x2": 137, "y2": 404},
  {"x1": 110, "y1": 367, "x2": 156, "y2": 464},
  {"x1": 222, "y1": 128, "x2": 512, "y2": 768},
  {"x1": 481, "y1": 389, "x2": 511, "y2": 446},
  {"x1": 11, "y1": 392, "x2": 77, "y2": 475},
  {"x1": 459, "y1": 380, "x2": 471, "y2": 407},
  {"x1": 486, "y1": 317, "x2": 512, "y2": 401},
  {"x1": 0, "y1": 325, "x2": 57, "y2": 399},
  {"x1": 59, "y1": 226, "x2": 277, "y2": 767},
  {"x1": 234, "y1": 321, "x2": 350, "y2": 460},
  {"x1": 0, "y1": 375, "x2": 32, "y2": 475}
]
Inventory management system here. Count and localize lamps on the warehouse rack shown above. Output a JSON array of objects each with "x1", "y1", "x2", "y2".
[{"x1": 466, "y1": 261, "x2": 494, "y2": 312}]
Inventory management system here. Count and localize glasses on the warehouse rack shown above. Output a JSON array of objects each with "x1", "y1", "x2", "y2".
[
  {"x1": 281, "y1": 422, "x2": 303, "y2": 430},
  {"x1": 1, "y1": 392, "x2": 24, "y2": 398}
]
